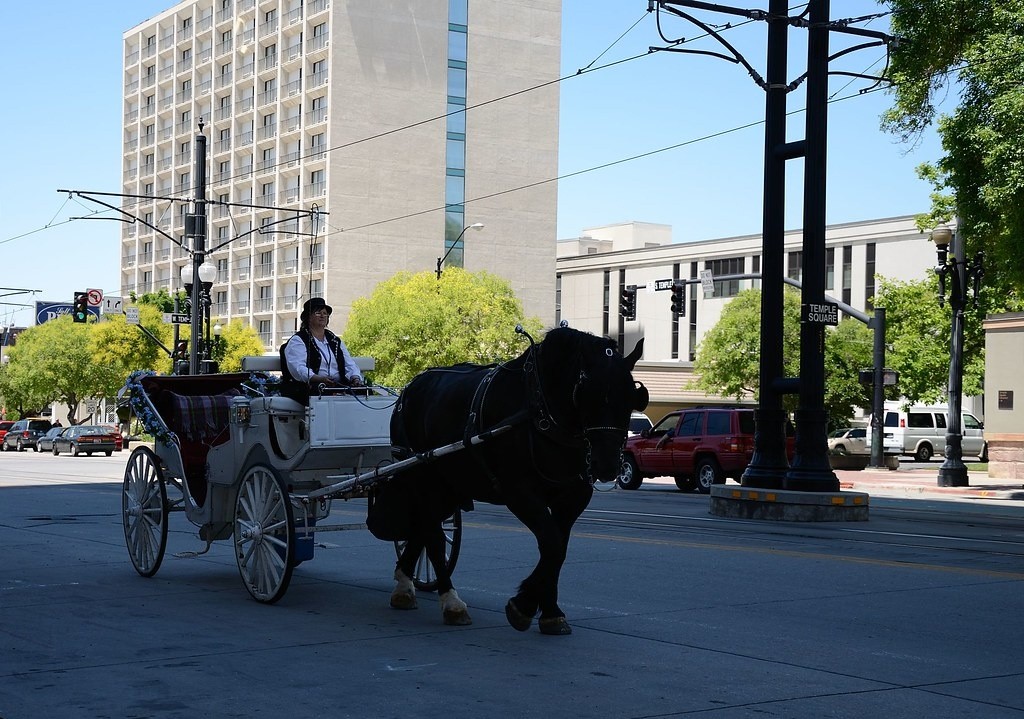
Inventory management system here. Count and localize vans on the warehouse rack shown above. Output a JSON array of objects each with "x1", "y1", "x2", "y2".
[
  {"x1": 621, "y1": 402, "x2": 796, "y2": 493},
  {"x1": 867, "y1": 406, "x2": 986, "y2": 463}
]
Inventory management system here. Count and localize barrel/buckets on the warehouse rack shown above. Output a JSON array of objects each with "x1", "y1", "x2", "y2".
[{"x1": 268, "y1": 517, "x2": 316, "y2": 567}]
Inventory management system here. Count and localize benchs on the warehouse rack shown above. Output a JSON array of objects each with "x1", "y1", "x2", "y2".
[
  {"x1": 244, "y1": 354, "x2": 374, "y2": 450},
  {"x1": 156, "y1": 387, "x2": 258, "y2": 476}
]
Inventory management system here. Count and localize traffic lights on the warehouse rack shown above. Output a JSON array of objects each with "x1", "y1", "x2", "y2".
[
  {"x1": 669, "y1": 280, "x2": 685, "y2": 318},
  {"x1": 621, "y1": 286, "x2": 637, "y2": 319},
  {"x1": 883, "y1": 371, "x2": 900, "y2": 386},
  {"x1": 73, "y1": 293, "x2": 88, "y2": 322},
  {"x1": 174, "y1": 339, "x2": 188, "y2": 359}
]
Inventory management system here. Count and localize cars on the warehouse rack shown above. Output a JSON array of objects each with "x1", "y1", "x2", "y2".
[
  {"x1": 827, "y1": 427, "x2": 870, "y2": 455},
  {"x1": 34, "y1": 425, "x2": 124, "y2": 455}
]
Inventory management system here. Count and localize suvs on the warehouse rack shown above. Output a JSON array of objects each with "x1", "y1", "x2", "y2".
[
  {"x1": 0, "y1": 417, "x2": 52, "y2": 452},
  {"x1": 629, "y1": 408, "x2": 655, "y2": 438}
]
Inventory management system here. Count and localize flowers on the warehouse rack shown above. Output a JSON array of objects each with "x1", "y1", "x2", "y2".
[
  {"x1": 240, "y1": 375, "x2": 287, "y2": 398},
  {"x1": 126, "y1": 369, "x2": 176, "y2": 449}
]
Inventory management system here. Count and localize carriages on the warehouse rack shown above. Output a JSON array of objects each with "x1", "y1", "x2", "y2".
[{"x1": 123, "y1": 325, "x2": 650, "y2": 635}]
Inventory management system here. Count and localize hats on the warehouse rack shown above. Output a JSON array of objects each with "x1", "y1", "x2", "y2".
[{"x1": 301, "y1": 298, "x2": 332, "y2": 321}]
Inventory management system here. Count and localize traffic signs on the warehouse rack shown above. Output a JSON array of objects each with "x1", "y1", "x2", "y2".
[
  {"x1": 104, "y1": 296, "x2": 122, "y2": 315},
  {"x1": 35, "y1": 300, "x2": 102, "y2": 327}
]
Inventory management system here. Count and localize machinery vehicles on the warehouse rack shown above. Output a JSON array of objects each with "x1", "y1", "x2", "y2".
[{"x1": 3, "y1": 309, "x2": 14, "y2": 346}]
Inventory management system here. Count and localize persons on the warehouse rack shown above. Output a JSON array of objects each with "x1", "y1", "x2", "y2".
[
  {"x1": 52, "y1": 420, "x2": 62, "y2": 427},
  {"x1": 655, "y1": 429, "x2": 674, "y2": 450},
  {"x1": 284, "y1": 298, "x2": 373, "y2": 406}
]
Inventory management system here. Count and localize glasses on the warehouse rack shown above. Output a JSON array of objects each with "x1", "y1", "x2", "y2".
[{"x1": 315, "y1": 310, "x2": 328, "y2": 317}]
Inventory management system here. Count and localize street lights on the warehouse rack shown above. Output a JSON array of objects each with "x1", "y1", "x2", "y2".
[
  {"x1": 436, "y1": 220, "x2": 486, "y2": 298},
  {"x1": 181, "y1": 261, "x2": 218, "y2": 372},
  {"x1": 933, "y1": 214, "x2": 985, "y2": 486}
]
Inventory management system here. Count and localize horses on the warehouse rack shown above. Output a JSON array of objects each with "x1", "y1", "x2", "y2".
[{"x1": 388, "y1": 327, "x2": 645, "y2": 638}]
